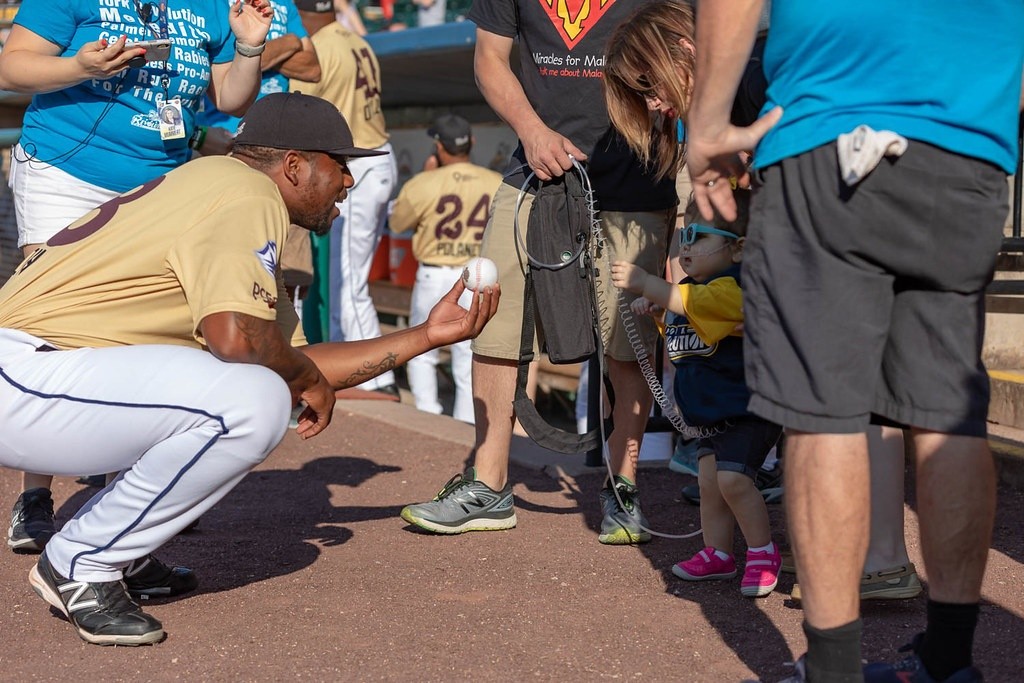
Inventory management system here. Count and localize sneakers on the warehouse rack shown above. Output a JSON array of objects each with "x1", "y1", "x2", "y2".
[
  {"x1": 684, "y1": 463, "x2": 783, "y2": 503},
  {"x1": 599, "y1": 472, "x2": 651, "y2": 544},
  {"x1": 672, "y1": 545, "x2": 737, "y2": 580},
  {"x1": 117, "y1": 553, "x2": 198, "y2": 601},
  {"x1": 863, "y1": 656, "x2": 930, "y2": 683},
  {"x1": 28, "y1": 548, "x2": 164, "y2": 645},
  {"x1": 7, "y1": 486, "x2": 57, "y2": 551},
  {"x1": 776, "y1": 652, "x2": 806, "y2": 683},
  {"x1": 400, "y1": 465, "x2": 517, "y2": 533},
  {"x1": 740, "y1": 545, "x2": 784, "y2": 596},
  {"x1": 791, "y1": 562, "x2": 922, "y2": 601}
]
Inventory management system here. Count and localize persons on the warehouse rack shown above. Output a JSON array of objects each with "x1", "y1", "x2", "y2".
[
  {"x1": 613, "y1": 189, "x2": 785, "y2": 599},
  {"x1": 0, "y1": 0, "x2": 923, "y2": 604},
  {"x1": 683, "y1": 0, "x2": 1024, "y2": 683},
  {"x1": 0, "y1": 90, "x2": 501, "y2": 645}
]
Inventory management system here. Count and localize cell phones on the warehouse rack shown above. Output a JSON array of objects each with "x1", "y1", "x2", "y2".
[{"x1": 100, "y1": 40, "x2": 171, "y2": 61}]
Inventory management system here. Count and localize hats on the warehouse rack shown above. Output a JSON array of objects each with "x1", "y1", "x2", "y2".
[
  {"x1": 234, "y1": 90, "x2": 389, "y2": 156},
  {"x1": 294, "y1": 0, "x2": 334, "y2": 12},
  {"x1": 426, "y1": 114, "x2": 471, "y2": 155}
]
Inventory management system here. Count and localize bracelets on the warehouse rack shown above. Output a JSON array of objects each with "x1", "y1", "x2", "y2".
[{"x1": 236, "y1": 39, "x2": 266, "y2": 57}]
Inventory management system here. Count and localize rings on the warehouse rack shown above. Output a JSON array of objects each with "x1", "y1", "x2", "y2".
[{"x1": 707, "y1": 180, "x2": 718, "y2": 186}]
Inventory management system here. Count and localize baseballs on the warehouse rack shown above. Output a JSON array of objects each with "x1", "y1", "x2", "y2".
[{"x1": 462, "y1": 257, "x2": 498, "y2": 293}]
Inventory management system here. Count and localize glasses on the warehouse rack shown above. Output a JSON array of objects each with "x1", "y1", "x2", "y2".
[
  {"x1": 678, "y1": 221, "x2": 739, "y2": 246},
  {"x1": 626, "y1": 74, "x2": 657, "y2": 99}
]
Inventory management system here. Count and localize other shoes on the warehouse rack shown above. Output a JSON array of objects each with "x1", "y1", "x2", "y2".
[
  {"x1": 373, "y1": 385, "x2": 401, "y2": 402},
  {"x1": 668, "y1": 435, "x2": 699, "y2": 478}
]
